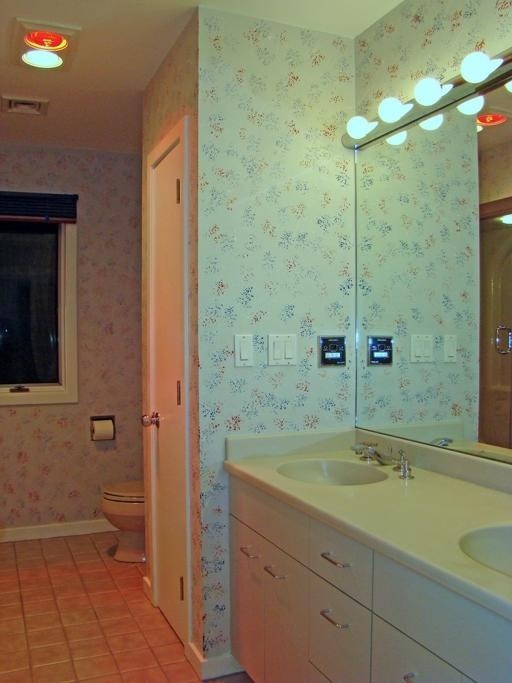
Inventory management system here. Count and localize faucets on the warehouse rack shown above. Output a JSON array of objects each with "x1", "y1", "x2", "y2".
[
  {"x1": 431, "y1": 437, "x2": 456, "y2": 449},
  {"x1": 355, "y1": 447, "x2": 393, "y2": 468}
]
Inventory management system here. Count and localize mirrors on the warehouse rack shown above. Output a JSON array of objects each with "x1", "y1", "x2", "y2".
[{"x1": 353, "y1": 73, "x2": 512, "y2": 467}]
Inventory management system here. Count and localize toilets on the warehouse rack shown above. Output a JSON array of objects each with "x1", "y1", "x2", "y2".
[{"x1": 99, "y1": 481, "x2": 148, "y2": 564}]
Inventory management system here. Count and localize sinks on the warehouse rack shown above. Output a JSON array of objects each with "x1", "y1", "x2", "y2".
[
  {"x1": 459, "y1": 524, "x2": 512, "y2": 575},
  {"x1": 275, "y1": 457, "x2": 388, "y2": 487}
]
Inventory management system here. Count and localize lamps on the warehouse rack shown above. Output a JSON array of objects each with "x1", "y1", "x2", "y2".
[
  {"x1": 346, "y1": 51, "x2": 504, "y2": 141},
  {"x1": 11, "y1": 17, "x2": 83, "y2": 72},
  {"x1": 386, "y1": 79, "x2": 512, "y2": 146}
]
